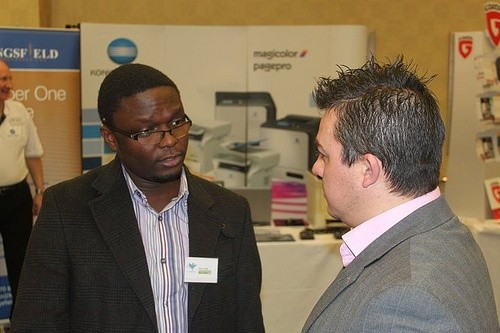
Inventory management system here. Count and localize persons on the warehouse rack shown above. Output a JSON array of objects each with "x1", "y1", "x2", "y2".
[
  {"x1": 0, "y1": 60, "x2": 46, "y2": 322},
  {"x1": 7, "y1": 62, "x2": 267, "y2": 333},
  {"x1": 299, "y1": 51, "x2": 500, "y2": 333}
]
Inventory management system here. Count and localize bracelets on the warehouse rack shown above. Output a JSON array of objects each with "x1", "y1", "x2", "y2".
[{"x1": 35, "y1": 189, "x2": 45, "y2": 195}]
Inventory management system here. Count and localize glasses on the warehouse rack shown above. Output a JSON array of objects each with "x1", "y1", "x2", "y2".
[{"x1": 111, "y1": 113, "x2": 192, "y2": 145}]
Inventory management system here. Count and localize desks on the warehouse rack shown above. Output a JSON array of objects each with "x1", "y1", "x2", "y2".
[{"x1": 255, "y1": 217, "x2": 500, "y2": 333}]
